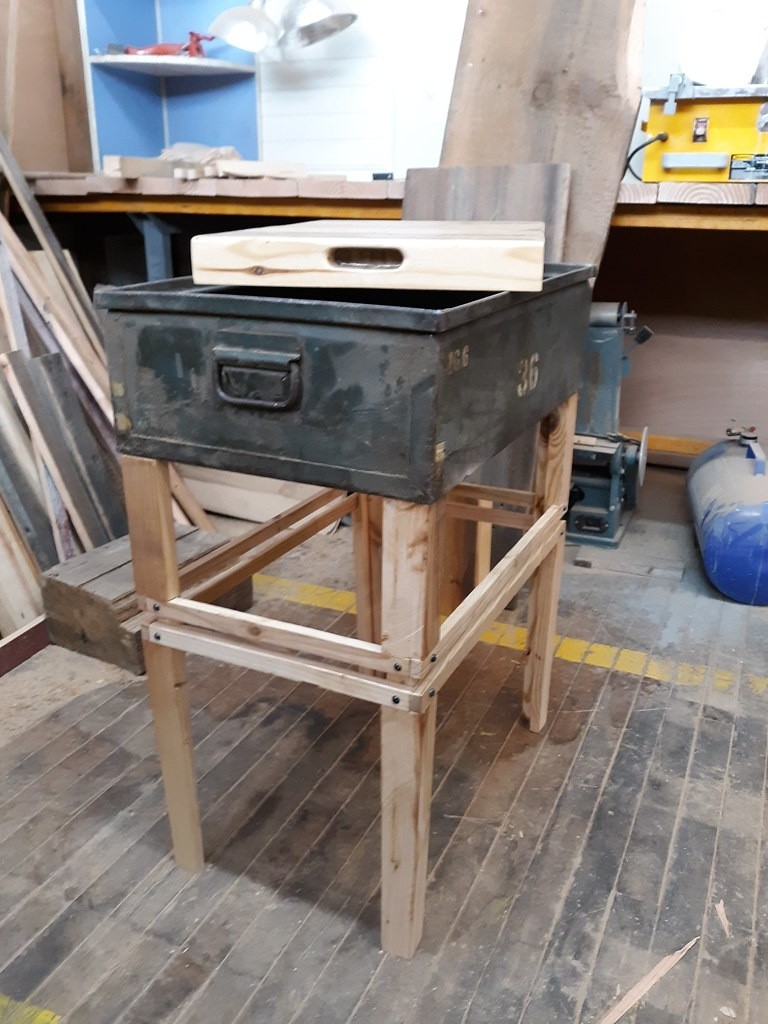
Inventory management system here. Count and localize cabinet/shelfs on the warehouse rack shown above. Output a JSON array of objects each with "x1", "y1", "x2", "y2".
[{"x1": 77, "y1": 0, "x2": 259, "y2": 281}]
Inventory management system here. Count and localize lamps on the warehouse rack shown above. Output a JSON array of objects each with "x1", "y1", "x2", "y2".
[{"x1": 207, "y1": 0, "x2": 358, "y2": 53}]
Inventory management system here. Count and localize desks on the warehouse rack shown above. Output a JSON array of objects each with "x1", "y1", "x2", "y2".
[{"x1": 32, "y1": 193, "x2": 768, "y2": 231}]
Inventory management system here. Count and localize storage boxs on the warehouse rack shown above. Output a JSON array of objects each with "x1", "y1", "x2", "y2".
[
  {"x1": 37, "y1": 523, "x2": 249, "y2": 677},
  {"x1": 93, "y1": 264, "x2": 600, "y2": 503}
]
